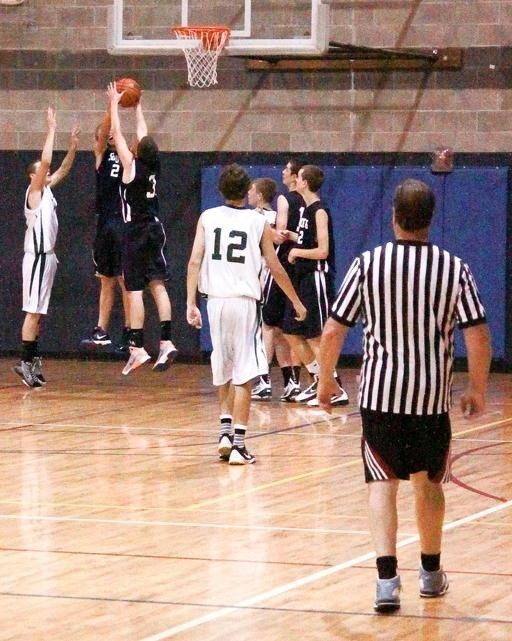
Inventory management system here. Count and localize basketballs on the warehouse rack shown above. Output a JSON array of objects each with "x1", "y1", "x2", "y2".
[{"x1": 115, "y1": 79, "x2": 139, "y2": 108}]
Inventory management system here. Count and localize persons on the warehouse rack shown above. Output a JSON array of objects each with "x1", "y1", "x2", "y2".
[
  {"x1": 106, "y1": 80, "x2": 179, "y2": 374},
  {"x1": 185, "y1": 162, "x2": 309, "y2": 468},
  {"x1": 252, "y1": 157, "x2": 308, "y2": 404},
  {"x1": 8, "y1": 105, "x2": 81, "y2": 391},
  {"x1": 278, "y1": 164, "x2": 350, "y2": 406},
  {"x1": 88, "y1": 97, "x2": 131, "y2": 352},
  {"x1": 315, "y1": 176, "x2": 493, "y2": 613},
  {"x1": 246, "y1": 175, "x2": 294, "y2": 404}
]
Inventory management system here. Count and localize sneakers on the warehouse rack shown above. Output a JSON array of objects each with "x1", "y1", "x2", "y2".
[
  {"x1": 153, "y1": 341, "x2": 178, "y2": 371},
  {"x1": 375, "y1": 572, "x2": 402, "y2": 609},
  {"x1": 11, "y1": 357, "x2": 47, "y2": 390},
  {"x1": 419, "y1": 570, "x2": 450, "y2": 597},
  {"x1": 121, "y1": 346, "x2": 151, "y2": 376},
  {"x1": 218, "y1": 435, "x2": 256, "y2": 464},
  {"x1": 81, "y1": 330, "x2": 114, "y2": 347},
  {"x1": 250, "y1": 377, "x2": 350, "y2": 406}
]
